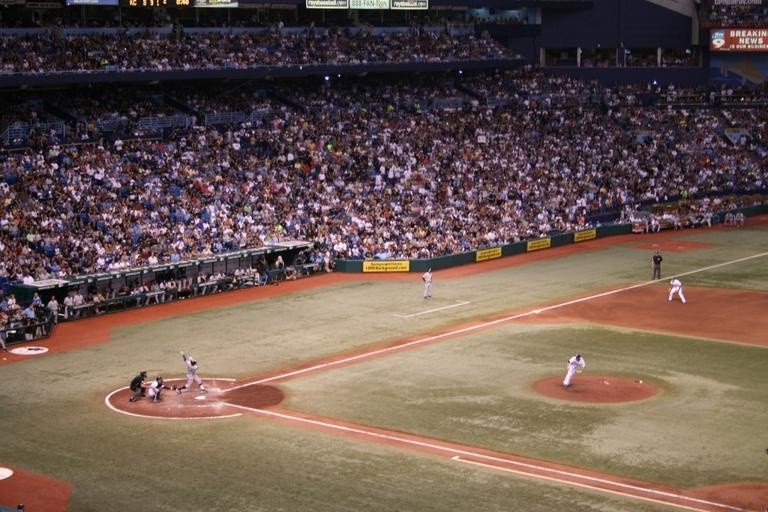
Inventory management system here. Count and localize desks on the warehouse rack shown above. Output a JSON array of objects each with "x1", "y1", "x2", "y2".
[{"x1": 72, "y1": 261, "x2": 317, "y2": 321}]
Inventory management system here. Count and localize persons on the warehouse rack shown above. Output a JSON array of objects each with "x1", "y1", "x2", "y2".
[
  {"x1": 129, "y1": 350, "x2": 207, "y2": 402},
  {"x1": 17, "y1": 504, "x2": 24, "y2": 512},
  {"x1": 1, "y1": 1, "x2": 767, "y2": 347},
  {"x1": 561, "y1": 354, "x2": 586, "y2": 387}
]
